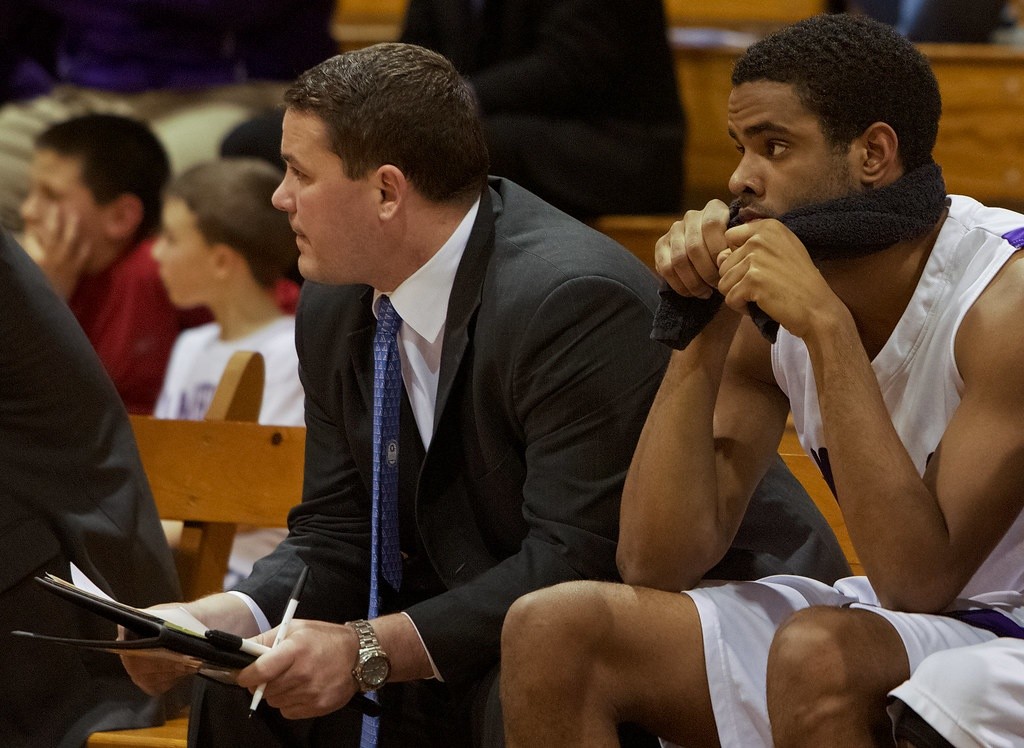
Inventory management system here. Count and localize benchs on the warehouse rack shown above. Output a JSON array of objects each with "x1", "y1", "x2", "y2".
[{"x1": 84, "y1": 350, "x2": 306, "y2": 748}]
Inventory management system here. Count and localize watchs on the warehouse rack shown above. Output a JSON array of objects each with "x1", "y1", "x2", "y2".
[{"x1": 344, "y1": 619, "x2": 392, "y2": 696}]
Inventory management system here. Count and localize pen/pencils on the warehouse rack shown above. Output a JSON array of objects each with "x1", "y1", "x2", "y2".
[
  {"x1": 245, "y1": 561, "x2": 312, "y2": 721},
  {"x1": 203, "y1": 627, "x2": 272, "y2": 658}
]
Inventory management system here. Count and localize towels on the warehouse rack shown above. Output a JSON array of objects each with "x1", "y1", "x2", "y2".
[{"x1": 649, "y1": 154, "x2": 948, "y2": 353}]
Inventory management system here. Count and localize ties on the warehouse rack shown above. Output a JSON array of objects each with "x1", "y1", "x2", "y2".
[{"x1": 358, "y1": 300, "x2": 402, "y2": 748}]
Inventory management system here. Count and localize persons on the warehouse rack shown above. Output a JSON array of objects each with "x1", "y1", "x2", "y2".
[
  {"x1": 117, "y1": 41, "x2": 853, "y2": 748},
  {"x1": 0, "y1": 0, "x2": 689, "y2": 288},
  {"x1": 498, "y1": 11, "x2": 1023, "y2": 748},
  {"x1": 149, "y1": 161, "x2": 306, "y2": 590},
  {"x1": 0, "y1": 76, "x2": 307, "y2": 748},
  {"x1": 824, "y1": 1, "x2": 1008, "y2": 43}
]
